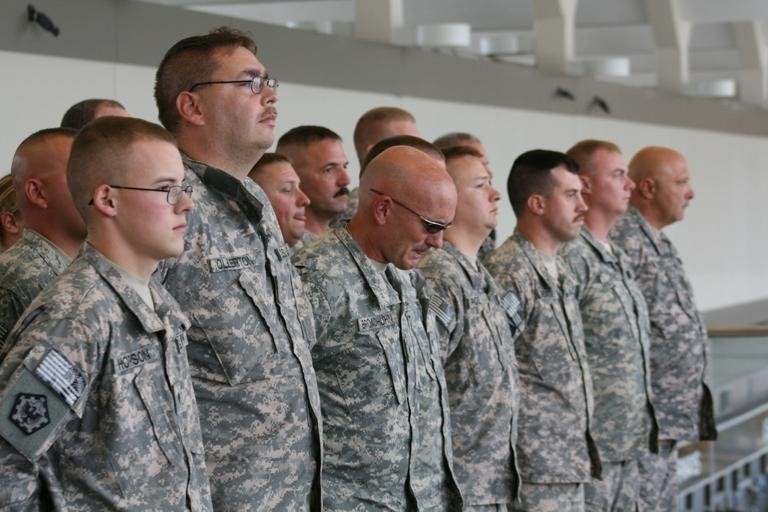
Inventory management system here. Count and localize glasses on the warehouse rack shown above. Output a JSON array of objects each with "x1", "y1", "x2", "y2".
[
  {"x1": 189, "y1": 75, "x2": 276, "y2": 95},
  {"x1": 88, "y1": 184, "x2": 193, "y2": 205},
  {"x1": 370, "y1": 188, "x2": 453, "y2": 234}
]
